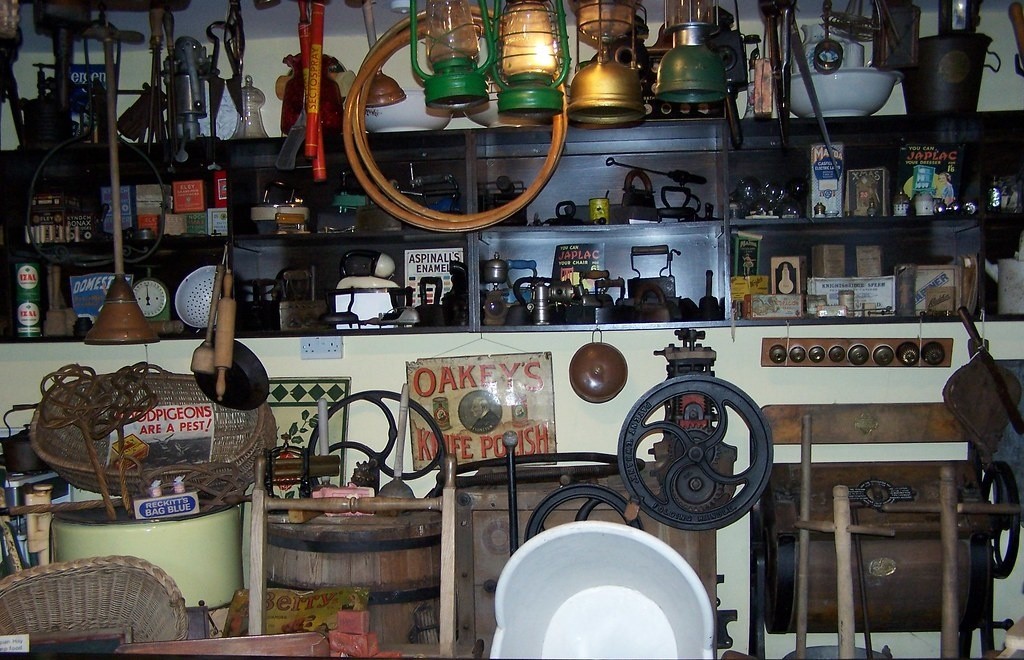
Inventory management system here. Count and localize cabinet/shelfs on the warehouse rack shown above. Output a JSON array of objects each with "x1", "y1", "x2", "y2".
[{"x1": 0, "y1": 109, "x2": 1024, "y2": 339}]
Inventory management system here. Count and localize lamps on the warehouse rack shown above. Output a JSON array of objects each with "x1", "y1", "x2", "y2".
[{"x1": 407, "y1": 0, "x2": 731, "y2": 127}]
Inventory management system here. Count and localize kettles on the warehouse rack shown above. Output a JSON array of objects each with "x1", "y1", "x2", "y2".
[{"x1": 2, "y1": 403, "x2": 45, "y2": 473}]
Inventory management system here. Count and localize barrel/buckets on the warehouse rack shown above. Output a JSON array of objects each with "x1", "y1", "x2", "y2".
[{"x1": 900, "y1": 33, "x2": 1001, "y2": 114}]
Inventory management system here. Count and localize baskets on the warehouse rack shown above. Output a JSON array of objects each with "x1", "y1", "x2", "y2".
[
  {"x1": 0, "y1": 555, "x2": 189, "y2": 642},
  {"x1": 30, "y1": 364, "x2": 277, "y2": 493}
]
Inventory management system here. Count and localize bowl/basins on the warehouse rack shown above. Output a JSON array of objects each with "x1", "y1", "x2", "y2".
[
  {"x1": 365, "y1": 89, "x2": 453, "y2": 133},
  {"x1": 463, "y1": 80, "x2": 571, "y2": 128},
  {"x1": 789, "y1": 67, "x2": 904, "y2": 118}
]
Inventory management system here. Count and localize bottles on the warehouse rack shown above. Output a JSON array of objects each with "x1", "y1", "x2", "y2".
[
  {"x1": 867, "y1": 202, "x2": 877, "y2": 217},
  {"x1": 839, "y1": 290, "x2": 854, "y2": 318},
  {"x1": 893, "y1": 189, "x2": 910, "y2": 216},
  {"x1": 986, "y1": 175, "x2": 1001, "y2": 213},
  {"x1": 814, "y1": 203, "x2": 826, "y2": 217}
]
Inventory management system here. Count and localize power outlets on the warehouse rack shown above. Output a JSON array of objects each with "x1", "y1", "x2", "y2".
[{"x1": 300, "y1": 335, "x2": 343, "y2": 361}]
